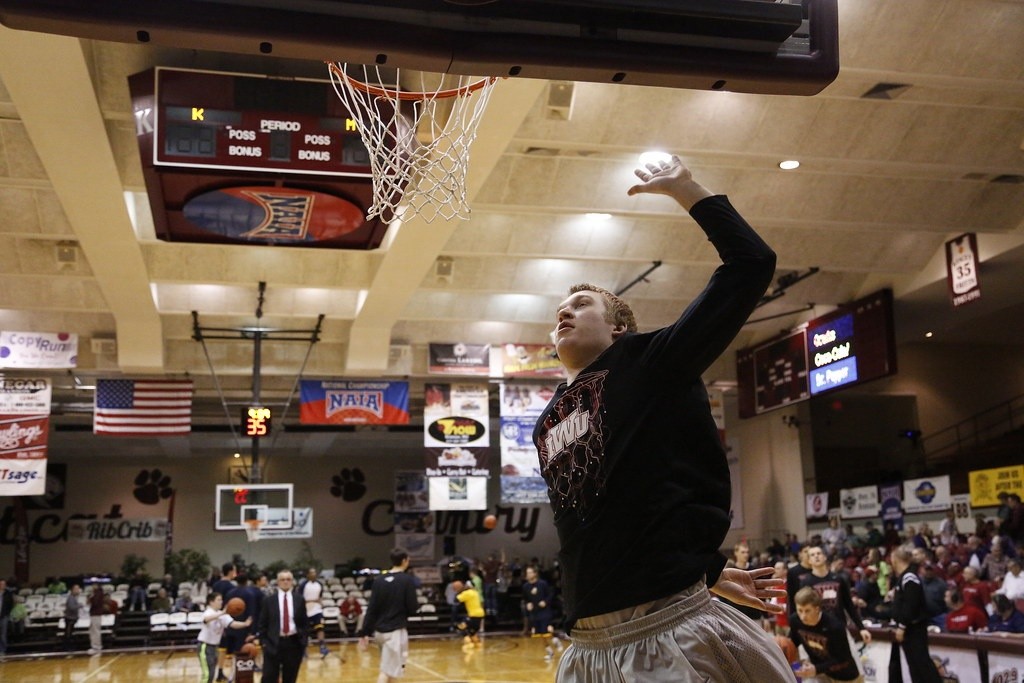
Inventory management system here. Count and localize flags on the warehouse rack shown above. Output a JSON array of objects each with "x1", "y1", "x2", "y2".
[
  {"x1": 299, "y1": 379, "x2": 409, "y2": 425},
  {"x1": 93, "y1": 379, "x2": 193, "y2": 437}
]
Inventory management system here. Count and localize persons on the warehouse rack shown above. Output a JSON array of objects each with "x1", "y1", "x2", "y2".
[
  {"x1": 716, "y1": 493, "x2": 1024, "y2": 683},
  {"x1": 1, "y1": 546, "x2": 563, "y2": 683},
  {"x1": 531, "y1": 156, "x2": 798, "y2": 683}
]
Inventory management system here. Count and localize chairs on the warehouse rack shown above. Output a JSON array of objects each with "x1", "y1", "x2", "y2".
[{"x1": 5, "y1": 567, "x2": 436, "y2": 637}]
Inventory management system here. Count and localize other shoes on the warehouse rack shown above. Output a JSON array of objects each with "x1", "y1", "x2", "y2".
[
  {"x1": 463, "y1": 642, "x2": 475, "y2": 649},
  {"x1": 87, "y1": 648, "x2": 102, "y2": 655},
  {"x1": 555, "y1": 640, "x2": 563, "y2": 652},
  {"x1": 545, "y1": 649, "x2": 554, "y2": 660},
  {"x1": 141, "y1": 603, "x2": 145, "y2": 611},
  {"x1": 320, "y1": 649, "x2": 328, "y2": 659},
  {"x1": 130, "y1": 605, "x2": 134, "y2": 611}
]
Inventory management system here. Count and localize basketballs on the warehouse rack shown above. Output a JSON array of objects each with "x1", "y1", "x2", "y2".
[
  {"x1": 484, "y1": 515, "x2": 497, "y2": 528},
  {"x1": 453, "y1": 580, "x2": 463, "y2": 591},
  {"x1": 241, "y1": 643, "x2": 257, "y2": 658},
  {"x1": 774, "y1": 636, "x2": 798, "y2": 664},
  {"x1": 228, "y1": 597, "x2": 246, "y2": 616}
]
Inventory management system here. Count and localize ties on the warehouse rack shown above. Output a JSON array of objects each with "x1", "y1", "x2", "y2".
[{"x1": 283, "y1": 593, "x2": 289, "y2": 633}]
